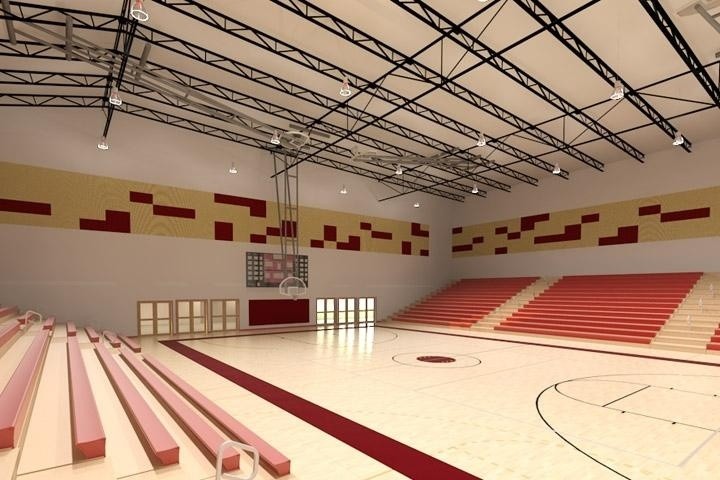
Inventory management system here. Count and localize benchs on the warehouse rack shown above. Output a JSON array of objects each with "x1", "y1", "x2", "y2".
[
  {"x1": 0, "y1": 293, "x2": 291, "y2": 480},
  {"x1": 388, "y1": 270, "x2": 719, "y2": 352}
]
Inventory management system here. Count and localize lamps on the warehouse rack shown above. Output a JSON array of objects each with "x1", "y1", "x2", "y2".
[
  {"x1": 271, "y1": 130, "x2": 281, "y2": 144},
  {"x1": 471, "y1": 181, "x2": 479, "y2": 193},
  {"x1": 229, "y1": 144, "x2": 238, "y2": 174},
  {"x1": 553, "y1": 162, "x2": 561, "y2": 175},
  {"x1": 395, "y1": 163, "x2": 402, "y2": 175},
  {"x1": 476, "y1": 131, "x2": 487, "y2": 146},
  {"x1": 673, "y1": 77, "x2": 684, "y2": 146},
  {"x1": 109, "y1": 86, "x2": 122, "y2": 105},
  {"x1": 610, "y1": 0, "x2": 624, "y2": 100},
  {"x1": 98, "y1": 136, "x2": 108, "y2": 150},
  {"x1": 340, "y1": 76, "x2": 351, "y2": 97},
  {"x1": 339, "y1": 171, "x2": 347, "y2": 194},
  {"x1": 130, "y1": 2, "x2": 150, "y2": 21}
]
